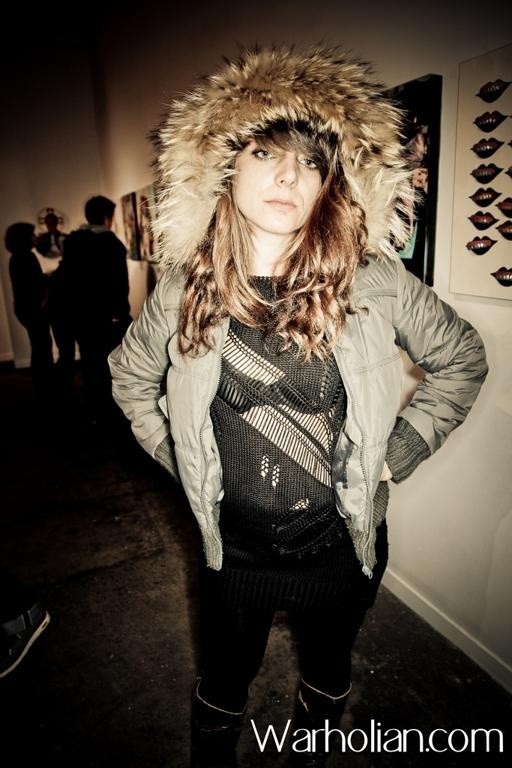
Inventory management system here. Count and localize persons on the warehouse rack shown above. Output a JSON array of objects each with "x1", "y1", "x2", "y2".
[
  {"x1": 5, "y1": 197, "x2": 132, "y2": 393},
  {"x1": 107, "y1": 40, "x2": 489, "y2": 767}
]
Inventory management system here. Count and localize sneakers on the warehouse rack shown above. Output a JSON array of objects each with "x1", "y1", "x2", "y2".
[{"x1": 0, "y1": 609, "x2": 50, "y2": 678}]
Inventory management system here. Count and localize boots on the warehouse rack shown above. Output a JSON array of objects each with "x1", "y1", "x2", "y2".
[
  {"x1": 294, "y1": 670, "x2": 352, "y2": 766},
  {"x1": 191, "y1": 681, "x2": 247, "y2": 768}
]
